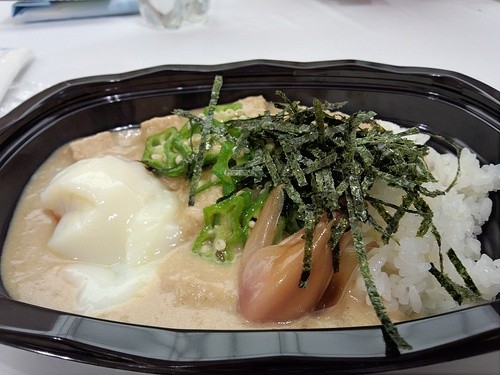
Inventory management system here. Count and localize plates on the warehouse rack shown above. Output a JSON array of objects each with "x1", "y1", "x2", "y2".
[{"x1": 0, "y1": 56, "x2": 498, "y2": 375}]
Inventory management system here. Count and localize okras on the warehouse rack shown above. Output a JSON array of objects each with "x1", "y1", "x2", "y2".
[{"x1": 140, "y1": 102, "x2": 286, "y2": 264}]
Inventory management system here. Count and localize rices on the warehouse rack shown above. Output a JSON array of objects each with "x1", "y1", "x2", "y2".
[{"x1": 354, "y1": 117, "x2": 500, "y2": 317}]
